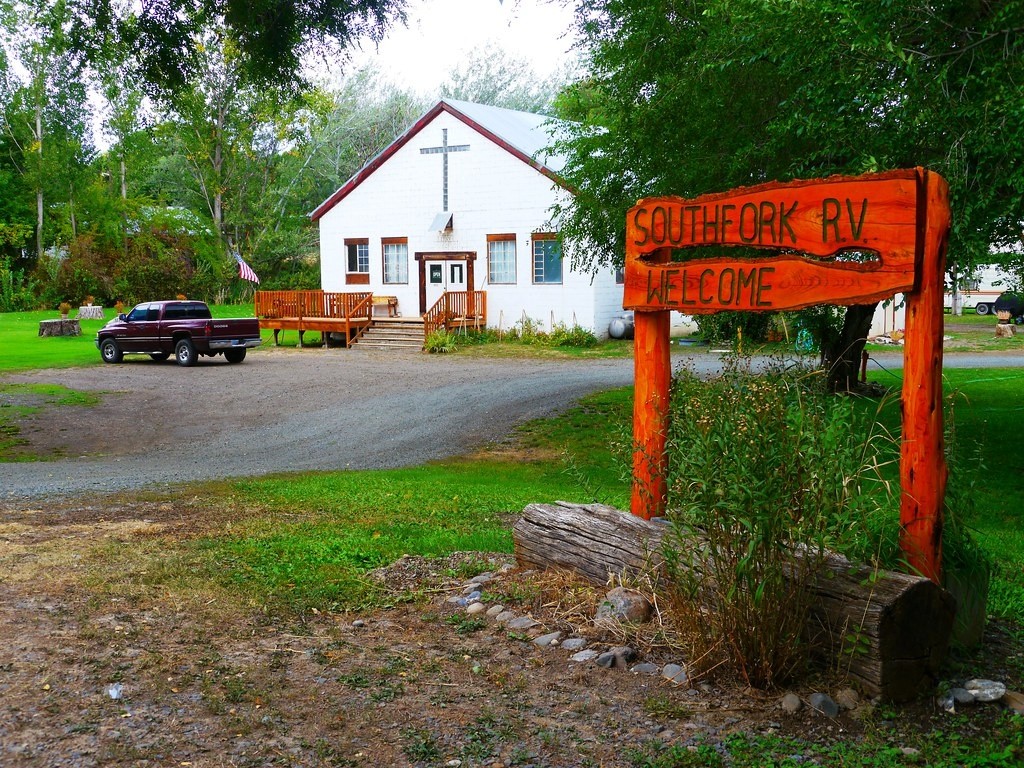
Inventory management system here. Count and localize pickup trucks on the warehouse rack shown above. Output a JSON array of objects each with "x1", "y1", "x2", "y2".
[{"x1": 95, "y1": 301, "x2": 262, "y2": 367}]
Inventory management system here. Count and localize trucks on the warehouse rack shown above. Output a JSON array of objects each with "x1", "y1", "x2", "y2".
[{"x1": 943, "y1": 278, "x2": 1002, "y2": 316}]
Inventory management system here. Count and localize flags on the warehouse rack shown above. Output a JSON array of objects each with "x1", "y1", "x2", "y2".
[{"x1": 234, "y1": 253, "x2": 259, "y2": 285}]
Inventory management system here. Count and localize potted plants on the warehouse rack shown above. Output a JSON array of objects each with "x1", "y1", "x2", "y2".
[
  {"x1": 126, "y1": 298, "x2": 130, "y2": 306},
  {"x1": 114, "y1": 301, "x2": 124, "y2": 316},
  {"x1": 58, "y1": 302, "x2": 72, "y2": 320},
  {"x1": 998, "y1": 311, "x2": 1011, "y2": 325},
  {"x1": 82, "y1": 300, "x2": 87, "y2": 306},
  {"x1": 890, "y1": 329, "x2": 904, "y2": 344},
  {"x1": 86, "y1": 295, "x2": 94, "y2": 307}
]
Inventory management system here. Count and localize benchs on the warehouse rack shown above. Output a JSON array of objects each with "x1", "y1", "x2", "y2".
[{"x1": 328, "y1": 295, "x2": 399, "y2": 318}]
